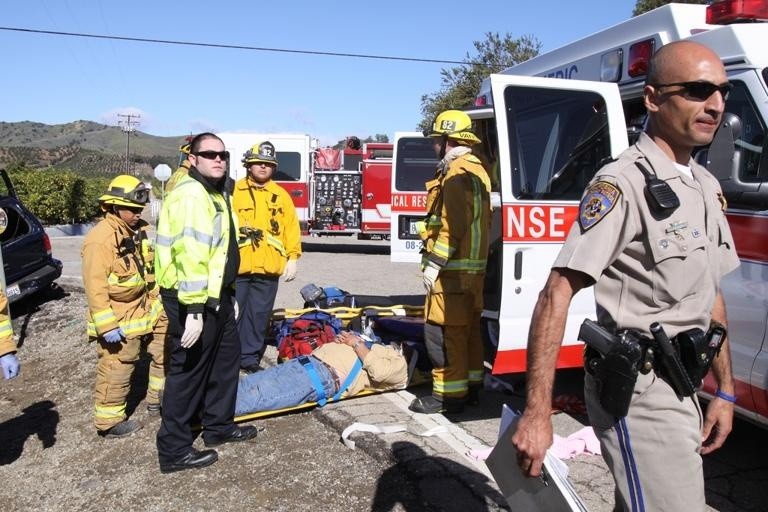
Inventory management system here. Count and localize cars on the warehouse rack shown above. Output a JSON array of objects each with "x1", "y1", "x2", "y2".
[{"x1": 0, "y1": 166, "x2": 63, "y2": 306}]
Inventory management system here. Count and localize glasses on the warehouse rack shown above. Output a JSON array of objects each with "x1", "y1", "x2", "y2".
[
  {"x1": 192, "y1": 151, "x2": 227, "y2": 161},
  {"x1": 652, "y1": 83, "x2": 735, "y2": 104}
]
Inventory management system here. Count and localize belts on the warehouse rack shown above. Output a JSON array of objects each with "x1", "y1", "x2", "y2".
[{"x1": 311, "y1": 354, "x2": 340, "y2": 393}]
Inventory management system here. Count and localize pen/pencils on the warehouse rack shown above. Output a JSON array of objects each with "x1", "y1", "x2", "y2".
[{"x1": 538, "y1": 467, "x2": 549, "y2": 487}]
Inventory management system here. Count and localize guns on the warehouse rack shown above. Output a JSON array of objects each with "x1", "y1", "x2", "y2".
[{"x1": 578, "y1": 318, "x2": 642, "y2": 417}]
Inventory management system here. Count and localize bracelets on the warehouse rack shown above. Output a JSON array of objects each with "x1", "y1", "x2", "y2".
[
  {"x1": 715, "y1": 391, "x2": 736, "y2": 403},
  {"x1": 352, "y1": 339, "x2": 362, "y2": 348}
]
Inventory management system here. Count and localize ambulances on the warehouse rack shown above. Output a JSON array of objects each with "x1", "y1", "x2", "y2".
[
  {"x1": 392, "y1": 1, "x2": 768, "y2": 432},
  {"x1": 210, "y1": 130, "x2": 395, "y2": 259}
]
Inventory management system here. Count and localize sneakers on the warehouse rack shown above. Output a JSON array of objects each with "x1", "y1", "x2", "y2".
[
  {"x1": 246, "y1": 363, "x2": 265, "y2": 375},
  {"x1": 146, "y1": 403, "x2": 162, "y2": 416},
  {"x1": 410, "y1": 395, "x2": 465, "y2": 415},
  {"x1": 466, "y1": 391, "x2": 481, "y2": 405},
  {"x1": 104, "y1": 420, "x2": 143, "y2": 438}
]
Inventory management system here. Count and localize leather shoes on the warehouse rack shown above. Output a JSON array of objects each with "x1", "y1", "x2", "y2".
[
  {"x1": 158, "y1": 448, "x2": 219, "y2": 474},
  {"x1": 203, "y1": 425, "x2": 257, "y2": 447}
]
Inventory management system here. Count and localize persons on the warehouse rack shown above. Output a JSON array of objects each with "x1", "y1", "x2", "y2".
[
  {"x1": 157, "y1": 329, "x2": 422, "y2": 412},
  {"x1": 410, "y1": 109, "x2": 491, "y2": 415},
  {"x1": 153, "y1": 132, "x2": 258, "y2": 474},
  {"x1": 163, "y1": 144, "x2": 196, "y2": 193},
  {"x1": 231, "y1": 141, "x2": 301, "y2": 376},
  {"x1": 511, "y1": 39, "x2": 736, "y2": 510},
  {"x1": 81, "y1": 174, "x2": 165, "y2": 439},
  {"x1": 0, "y1": 247, "x2": 19, "y2": 380}
]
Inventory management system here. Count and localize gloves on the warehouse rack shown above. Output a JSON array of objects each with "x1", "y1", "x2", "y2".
[
  {"x1": 102, "y1": 327, "x2": 127, "y2": 344},
  {"x1": 179, "y1": 313, "x2": 204, "y2": 349},
  {"x1": 230, "y1": 294, "x2": 240, "y2": 321},
  {"x1": 422, "y1": 259, "x2": 442, "y2": 293},
  {"x1": 0, "y1": 353, "x2": 20, "y2": 379},
  {"x1": 282, "y1": 257, "x2": 299, "y2": 283}
]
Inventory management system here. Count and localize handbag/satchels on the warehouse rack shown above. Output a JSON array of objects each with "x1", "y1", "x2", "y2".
[
  {"x1": 276, "y1": 318, "x2": 337, "y2": 364},
  {"x1": 277, "y1": 310, "x2": 342, "y2": 349}
]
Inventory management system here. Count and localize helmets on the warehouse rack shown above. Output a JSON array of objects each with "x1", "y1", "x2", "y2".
[
  {"x1": 97, "y1": 174, "x2": 151, "y2": 214},
  {"x1": 428, "y1": 110, "x2": 482, "y2": 147},
  {"x1": 242, "y1": 141, "x2": 279, "y2": 168}
]
